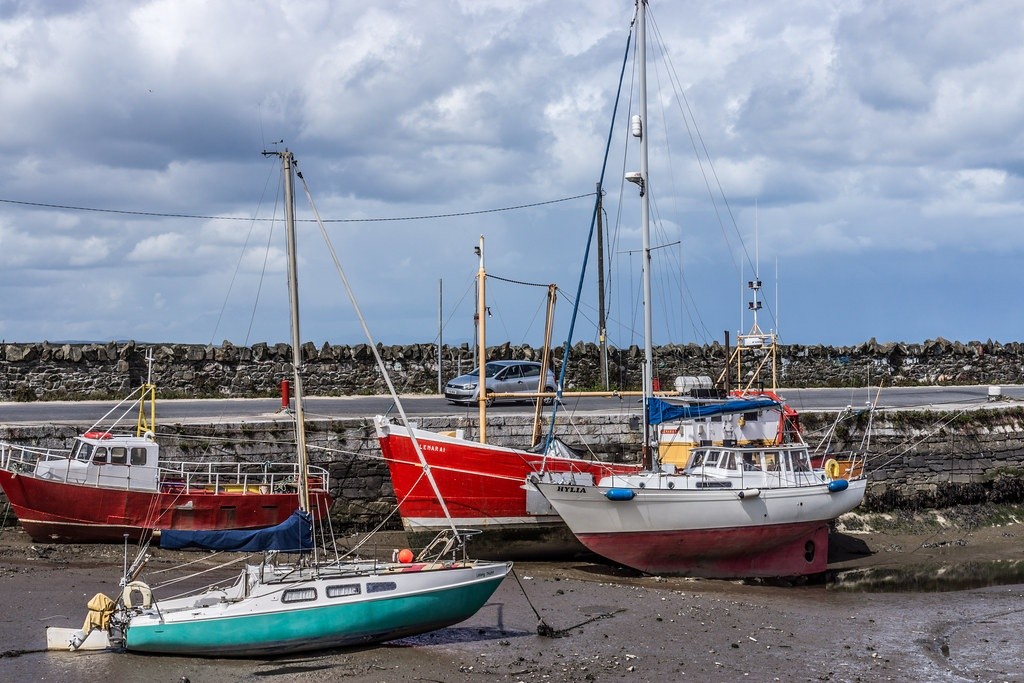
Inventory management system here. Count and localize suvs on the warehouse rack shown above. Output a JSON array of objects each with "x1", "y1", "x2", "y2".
[{"x1": 444, "y1": 359, "x2": 558, "y2": 408}]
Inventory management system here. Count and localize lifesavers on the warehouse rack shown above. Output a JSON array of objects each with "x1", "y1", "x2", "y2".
[
  {"x1": 84, "y1": 430, "x2": 113, "y2": 440},
  {"x1": 824, "y1": 458, "x2": 840, "y2": 479},
  {"x1": 121, "y1": 580, "x2": 153, "y2": 610}
]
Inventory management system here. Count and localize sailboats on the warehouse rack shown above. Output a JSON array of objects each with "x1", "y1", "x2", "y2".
[
  {"x1": 372, "y1": 8, "x2": 838, "y2": 571},
  {"x1": 524, "y1": 0, "x2": 868, "y2": 581},
  {"x1": 67, "y1": 137, "x2": 515, "y2": 661}
]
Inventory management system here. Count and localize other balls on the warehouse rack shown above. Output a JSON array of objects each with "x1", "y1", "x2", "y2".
[{"x1": 398, "y1": 549, "x2": 415, "y2": 563}]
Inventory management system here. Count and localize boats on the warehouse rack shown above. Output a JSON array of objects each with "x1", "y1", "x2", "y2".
[{"x1": 0, "y1": 349, "x2": 336, "y2": 549}]
